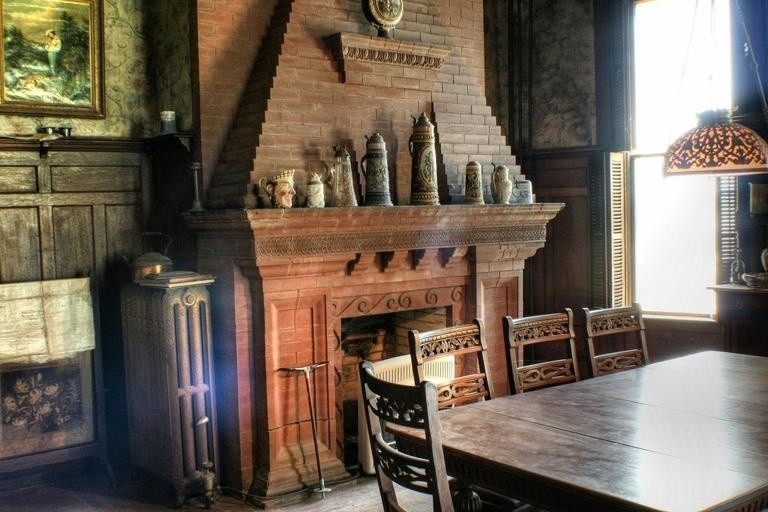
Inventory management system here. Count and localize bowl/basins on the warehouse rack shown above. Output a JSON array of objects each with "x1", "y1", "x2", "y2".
[
  {"x1": 741, "y1": 272, "x2": 768, "y2": 290},
  {"x1": 37, "y1": 126, "x2": 73, "y2": 138}
]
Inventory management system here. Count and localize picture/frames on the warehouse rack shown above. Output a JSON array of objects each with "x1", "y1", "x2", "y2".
[{"x1": 0, "y1": 0, "x2": 109, "y2": 120}]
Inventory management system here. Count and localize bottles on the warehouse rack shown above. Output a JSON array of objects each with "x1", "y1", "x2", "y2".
[
  {"x1": 304, "y1": 171, "x2": 326, "y2": 208},
  {"x1": 407, "y1": 112, "x2": 440, "y2": 206},
  {"x1": 359, "y1": 132, "x2": 393, "y2": 206},
  {"x1": 331, "y1": 144, "x2": 359, "y2": 207},
  {"x1": 728, "y1": 248, "x2": 746, "y2": 283},
  {"x1": 464, "y1": 162, "x2": 486, "y2": 206}
]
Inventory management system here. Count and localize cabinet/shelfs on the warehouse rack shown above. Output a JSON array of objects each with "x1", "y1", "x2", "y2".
[{"x1": 705, "y1": 281, "x2": 768, "y2": 355}]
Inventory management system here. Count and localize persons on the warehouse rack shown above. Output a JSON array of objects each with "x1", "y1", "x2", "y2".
[{"x1": 43, "y1": 31, "x2": 62, "y2": 75}]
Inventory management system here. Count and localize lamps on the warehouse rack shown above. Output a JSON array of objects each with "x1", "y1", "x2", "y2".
[{"x1": 662, "y1": 1, "x2": 768, "y2": 180}]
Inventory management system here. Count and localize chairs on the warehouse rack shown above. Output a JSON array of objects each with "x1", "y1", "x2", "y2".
[
  {"x1": 356, "y1": 358, "x2": 529, "y2": 511},
  {"x1": 406, "y1": 316, "x2": 523, "y2": 509},
  {"x1": 581, "y1": 301, "x2": 650, "y2": 376},
  {"x1": 501, "y1": 306, "x2": 580, "y2": 396}
]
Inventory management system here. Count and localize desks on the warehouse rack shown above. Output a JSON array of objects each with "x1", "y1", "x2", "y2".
[{"x1": 384, "y1": 347, "x2": 768, "y2": 510}]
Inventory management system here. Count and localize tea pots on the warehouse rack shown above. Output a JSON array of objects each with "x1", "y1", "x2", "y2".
[
  {"x1": 489, "y1": 161, "x2": 512, "y2": 206},
  {"x1": 120, "y1": 230, "x2": 174, "y2": 282}
]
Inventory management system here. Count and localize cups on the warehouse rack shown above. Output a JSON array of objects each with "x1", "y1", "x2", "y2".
[
  {"x1": 512, "y1": 180, "x2": 534, "y2": 205},
  {"x1": 159, "y1": 110, "x2": 177, "y2": 136},
  {"x1": 257, "y1": 176, "x2": 294, "y2": 209}
]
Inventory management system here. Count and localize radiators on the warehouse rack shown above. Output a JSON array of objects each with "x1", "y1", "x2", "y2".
[{"x1": 352, "y1": 346, "x2": 458, "y2": 479}]
